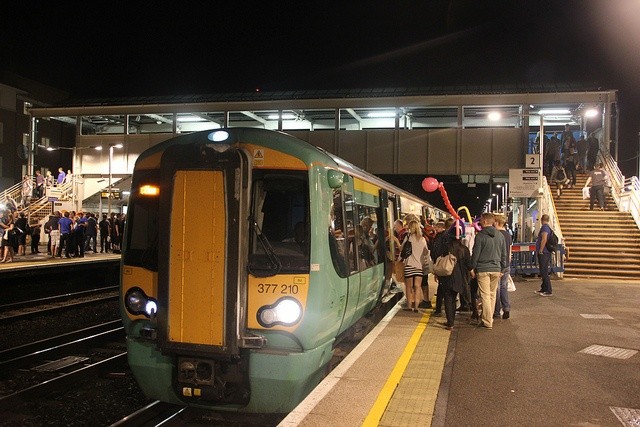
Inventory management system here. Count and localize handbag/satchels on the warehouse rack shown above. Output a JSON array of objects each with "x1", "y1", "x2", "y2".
[
  {"x1": 582, "y1": 185, "x2": 589, "y2": 199},
  {"x1": 433, "y1": 251, "x2": 457, "y2": 276},
  {"x1": 401, "y1": 234, "x2": 412, "y2": 259}
]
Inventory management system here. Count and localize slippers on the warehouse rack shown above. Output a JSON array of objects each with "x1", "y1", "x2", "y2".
[
  {"x1": 403, "y1": 308, "x2": 412, "y2": 311},
  {"x1": 414, "y1": 309, "x2": 419, "y2": 312}
]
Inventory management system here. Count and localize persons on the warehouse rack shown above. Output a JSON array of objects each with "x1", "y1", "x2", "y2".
[
  {"x1": 30, "y1": 215, "x2": 42, "y2": 255},
  {"x1": 585, "y1": 163, "x2": 605, "y2": 210},
  {"x1": 351, "y1": 225, "x2": 377, "y2": 270},
  {"x1": 360, "y1": 215, "x2": 382, "y2": 262},
  {"x1": 0, "y1": 210, "x2": 9, "y2": 259},
  {"x1": 599, "y1": 163, "x2": 611, "y2": 198},
  {"x1": 57, "y1": 168, "x2": 65, "y2": 183},
  {"x1": 45, "y1": 211, "x2": 123, "y2": 258},
  {"x1": 393, "y1": 214, "x2": 472, "y2": 329},
  {"x1": 67, "y1": 170, "x2": 72, "y2": 181},
  {"x1": 7, "y1": 195, "x2": 16, "y2": 209},
  {"x1": 281, "y1": 222, "x2": 309, "y2": 255},
  {"x1": 35, "y1": 170, "x2": 45, "y2": 198},
  {"x1": 1, "y1": 214, "x2": 14, "y2": 263},
  {"x1": 15, "y1": 212, "x2": 28, "y2": 255},
  {"x1": 534, "y1": 214, "x2": 553, "y2": 297},
  {"x1": 21, "y1": 174, "x2": 33, "y2": 206},
  {"x1": 544, "y1": 124, "x2": 599, "y2": 196},
  {"x1": 472, "y1": 213, "x2": 507, "y2": 330},
  {"x1": 493, "y1": 215, "x2": 510, "y2": 319},
  {"x1": 46, "y1": 171, "x2": 53, "y2": 186}
]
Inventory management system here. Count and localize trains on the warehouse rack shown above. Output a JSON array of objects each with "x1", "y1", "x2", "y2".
[{"x1": 118, "y1": 126, "x2": 470, "y2": 418}]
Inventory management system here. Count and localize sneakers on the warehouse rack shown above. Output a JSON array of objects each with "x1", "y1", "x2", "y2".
[
  {"x1": 419, "y1": 299, "x2": 432, "y2": 309},
  {"x1": 534, "y1": 291, "x2": 542, "y2": 294},
  {"x1": 540, "y1": 291, "x2": 552, "y2": 296},
  {"x1": 502, "y1": 312, "x2": 509, "y2": 319}
]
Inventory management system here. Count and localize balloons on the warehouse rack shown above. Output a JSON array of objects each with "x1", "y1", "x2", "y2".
[{"x1": 419, "y1": 177, "x2": 458, "y2": 221}]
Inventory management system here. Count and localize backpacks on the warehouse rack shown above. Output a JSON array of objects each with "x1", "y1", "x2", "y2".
[
  {"x1": 544, "y1": 225, "x2": 558, "y2": 253},
  {"x1": 557, "y1": 166, "x2": 565, "y2": 180}
]
